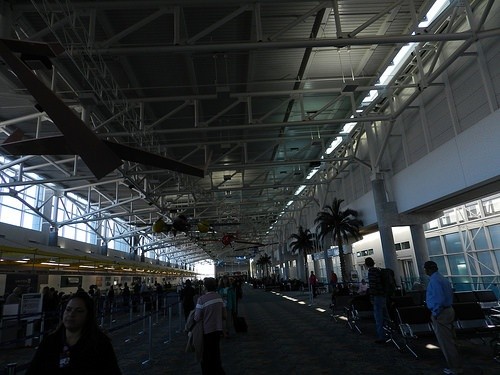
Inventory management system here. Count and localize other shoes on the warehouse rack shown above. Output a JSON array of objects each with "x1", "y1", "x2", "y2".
[
  {"x1": 443, "y1": 368, "x2": 464, "y2": 375},
  {"x1": 375, "y1": 340, "x2": 385, "y2": 344}
]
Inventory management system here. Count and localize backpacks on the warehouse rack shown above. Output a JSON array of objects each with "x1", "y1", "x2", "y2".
[{"x1": 381, "y1": 268, "x2": 396, "y2": 296}]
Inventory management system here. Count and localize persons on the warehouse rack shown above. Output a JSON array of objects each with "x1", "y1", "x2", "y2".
[
  {"x1": 365, "y1": 257, "x2": 387, "y2": 350},
  {"x1": 179, "y1": 275, "x2": 243, "y2": 375},
  {"x1": 424, "y1": 260, "x2": 463, "y2": 375},
  {"x1": 42, "y1": 280, "x2": 172, "y2": 317},
  {"x1": 26, "y1": 293, "x2": 123, "y2": 375},
  {"x1": 309, "y1": 271, "x2": 316, "y2": 299},
  {"x1": 330, "y1": 271, "x2": 338, "y2": 298}
]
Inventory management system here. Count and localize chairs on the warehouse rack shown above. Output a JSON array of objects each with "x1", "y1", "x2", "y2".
[{"x1": 330, "y1": 289, "x2": 500, "y2": 358}]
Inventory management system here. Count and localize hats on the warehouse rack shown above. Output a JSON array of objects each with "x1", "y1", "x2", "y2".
[{"x1": 204, "y1": 278, "x2": 217, "y2": 290}]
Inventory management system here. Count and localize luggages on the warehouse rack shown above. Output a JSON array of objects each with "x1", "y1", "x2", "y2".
[{"x1": 233, "y1": 317, "x2": 248, "y2": 333}]
